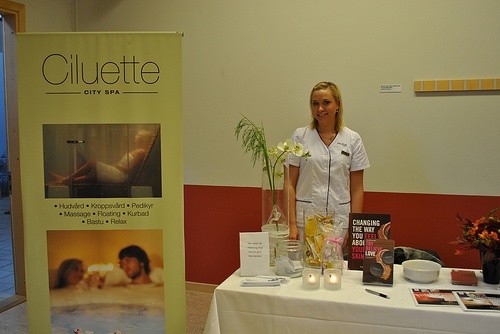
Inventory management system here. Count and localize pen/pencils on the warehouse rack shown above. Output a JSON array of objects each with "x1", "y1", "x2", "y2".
[{"x1": 365, "y1": 289, "x2": 390, "y2": 299}]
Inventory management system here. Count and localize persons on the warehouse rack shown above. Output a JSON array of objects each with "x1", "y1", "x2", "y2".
[
  {"x1": 54, "y1": 259, "x2": 100, "y2": 288},
  {"x1": 282, "y1": 81, "x2": 372, "y2": 258},
  {"x1": 100, "y1": 245, "x2": 153, "y2": 285},
  {"x1": 50, "y1": 129, "x2": 152, "y2": 184}
]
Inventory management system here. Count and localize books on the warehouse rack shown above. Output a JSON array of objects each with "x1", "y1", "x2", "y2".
[
  {"x1": 348, "y1": 213, "x2": 391, "y2": 271},
  {"x1": 362, "y1": 239, "x2": 395, "y2": 287}
]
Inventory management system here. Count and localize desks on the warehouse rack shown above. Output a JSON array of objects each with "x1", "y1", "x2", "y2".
[{"x1": 201, "y1": 259, "x2": 500, "y2": 334}]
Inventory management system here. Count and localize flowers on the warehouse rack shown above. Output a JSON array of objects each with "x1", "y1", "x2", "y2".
[
  {"x1": 444, "y1": 205, "x2": 500, "y2": 267},
  {"x1": 266, "y1": 138, "x2": 312, "y2": 258}
]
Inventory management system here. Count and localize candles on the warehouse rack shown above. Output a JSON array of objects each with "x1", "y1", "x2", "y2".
[
  {"x1": 302, "y1": 267, "x2": 321, "y2": 291},
  {"x1": 322, "y1": 268, "x2": 342, "y2": 290}
]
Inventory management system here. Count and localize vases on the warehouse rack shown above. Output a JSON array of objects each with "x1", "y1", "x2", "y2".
[
  {"x1": 478, "y1": 242, "x2": 500, "y2": 286},
  {"x1": 263, "y1": 222, "x2": 290, "y2": 267}
]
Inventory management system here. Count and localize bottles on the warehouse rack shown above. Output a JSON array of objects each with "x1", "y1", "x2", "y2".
[{"x1": 320, "y1": 218, "x2": 345, "y2": 277}]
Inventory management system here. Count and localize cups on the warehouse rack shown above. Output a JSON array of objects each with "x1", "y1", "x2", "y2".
[
  {"x1": 274, "y1": 240, "x2": 305, "y2": 277},
  {"x1": 302, "y1": 267, "x2": 321, "y2": 290},
  {"x1": 323, "y1": 268, "x2": 342, "y2": 290},
  {"x1": 302, "y1": 207, "x2": 335, "y2": 269}
]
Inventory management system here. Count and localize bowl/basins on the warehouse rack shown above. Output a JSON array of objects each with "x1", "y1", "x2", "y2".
[{"x1": 402, "y1": 260, "x2": 441, "y2": 282}]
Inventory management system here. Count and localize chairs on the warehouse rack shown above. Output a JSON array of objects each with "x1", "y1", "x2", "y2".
[{"x1": 393, "y1": 246, "x2": 442, "y2": 266}]
